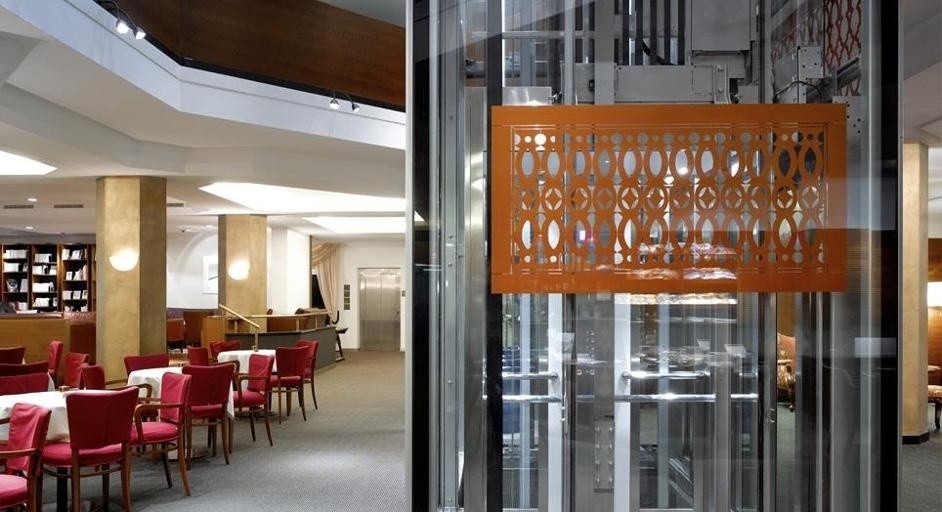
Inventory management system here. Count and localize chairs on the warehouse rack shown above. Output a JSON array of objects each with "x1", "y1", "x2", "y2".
[
  {"x1": 1, "y1": 340, "x2": 242, "y2": 398},
  {"x1": 178, "y1": 363, "x2": 238, "y2": 466},
  {"x1": 41, "y1": 388, "x2": 140, "y2": 512},
  {"x1": 0, "y1": 403, "x2": 53, "y2": 512},
  {"x1": 268, "y1": 345, "x2": 312, "y2": 425},
  {"x1": 224, "y1": 354, "x2": 276, "y2": 453},
  {"x1": 271, "y1": 341, "x2": 320, "y2": 413},
  {"x1": 167, "y1": 307, "x2": 215, "y2": 351},
  {"x1": 120, "y1": 371, "x2": 193, "y2": 499}
]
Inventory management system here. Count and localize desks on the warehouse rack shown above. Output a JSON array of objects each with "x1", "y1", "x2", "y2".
[
  {"x1": 0, "y1": 390, "x2": 119, "y2": 512},
  {"x1": 126, "y1": 367, "x2": 235, "y2": 458},
  {"x1": 218, "y1": 348, "x2": 278, "y2": 420}
]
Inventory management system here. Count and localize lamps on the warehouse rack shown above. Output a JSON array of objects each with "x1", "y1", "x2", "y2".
[
  {"x1": 99, "y1": 0, "x2": 146, "y2": 40},
  {"x1": 329, "y1": 88, "x2": 361, "y2": 112}
]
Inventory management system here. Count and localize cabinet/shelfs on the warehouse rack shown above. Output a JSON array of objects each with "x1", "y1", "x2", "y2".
[{"x1": 0, "y1": 243, "x2": 96, "y2": 312}]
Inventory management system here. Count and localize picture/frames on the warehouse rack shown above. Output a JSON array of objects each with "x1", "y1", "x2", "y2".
[{"x1": 201, "y1": 254, "x2": 219, "y2": 294}]
